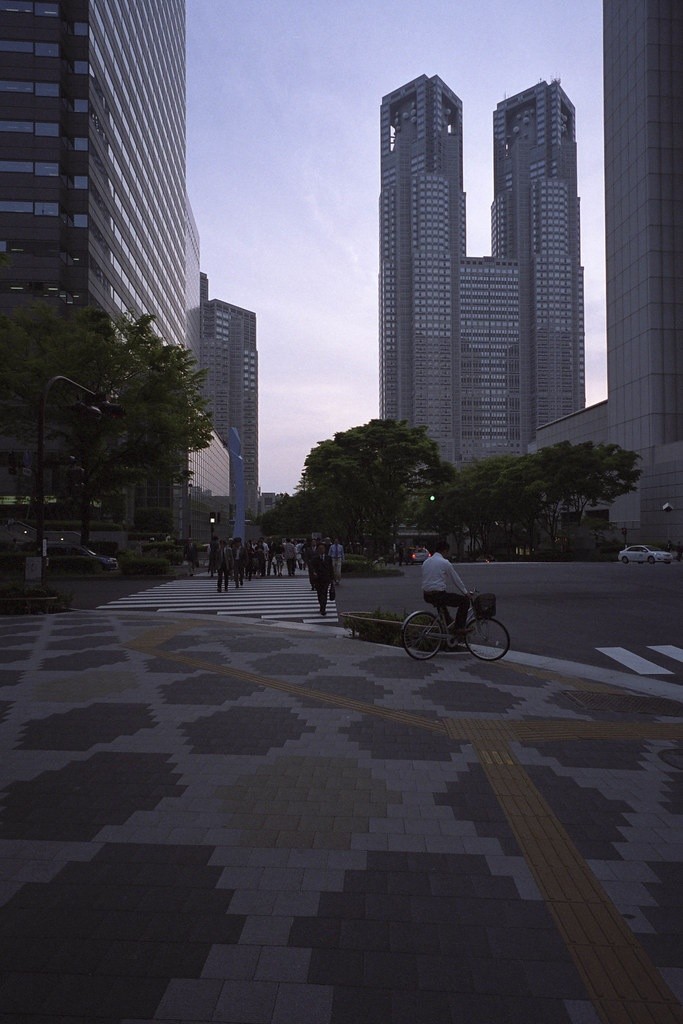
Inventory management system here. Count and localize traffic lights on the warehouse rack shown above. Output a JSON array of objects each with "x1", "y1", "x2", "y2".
[
  {"x1": 622, "y1": 527, "x2": 627, "y2": 535},
  {"x1": 209, "y1": 512, "x2": 216, "y2": 524},
  {"x1": 99, "y1": 398, "x2": 128, "y2": 419},
  {"x1": 429, "y1": 495, "x2": 448, "y2": 501}
]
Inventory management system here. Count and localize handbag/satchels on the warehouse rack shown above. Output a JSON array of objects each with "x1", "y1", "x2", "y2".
[{"x1": 329, "y1": 584, "x2": 336, "y2": 601}]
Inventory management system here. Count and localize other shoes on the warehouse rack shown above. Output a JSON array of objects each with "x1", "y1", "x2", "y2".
[
  {"x1": 236, "y1": 584, "x2": 239, "y2": 588},
  {"x1": 455, "y1": 628, "x2": 467, "y2": 635},
  {"x1": 240, "y1": 580, "x2": 243, "y2": 586},
  {"x1": 278, "y1": 572, "x2": 282, "y2": 577},
  {"x1": 217, "y1": 588, "x2": 221, "y2": 592},
  {"x1": 320, "y1": 608, "x2": 326, "y2": 616},
  {"x1": 288, "y1": 572, "x2": 295, "y2": 576},
  {"x1": 225, "y1": 586, "x2": 227, "y2": 591}
]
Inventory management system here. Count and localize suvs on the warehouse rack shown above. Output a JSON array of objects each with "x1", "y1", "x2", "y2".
[{"x1": 18, "y1": 543, "x2": 118, "y2": 574}]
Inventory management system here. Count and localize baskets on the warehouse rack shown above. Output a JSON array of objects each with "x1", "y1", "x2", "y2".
[{"x1": 475, "y1": 593, "x2": 497, "y2": 618}]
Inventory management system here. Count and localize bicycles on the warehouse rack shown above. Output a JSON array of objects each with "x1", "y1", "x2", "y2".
[{"x1": 400, "y1": 583, "x2": 511, "y2": 661}]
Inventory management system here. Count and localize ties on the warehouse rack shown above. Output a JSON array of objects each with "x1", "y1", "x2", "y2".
[
  {"x1": 335, "y1": 546, "x2": 338, "y2": 560},
  {"x1": 222, "y1": 550, "x2": 225, "y2": 561},
  {"x1": 321, "y1": 554, "x2": 324, "y2": 561}
]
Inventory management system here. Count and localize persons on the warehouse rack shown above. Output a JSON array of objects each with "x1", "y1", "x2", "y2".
[
  {"x1": 422, "y1": 543, "x2": 475, "y2": 645},
  {"x1": 184, "y1": 539, "x2": 199, "y2": 578},
  {"x1": 398, "y1": 545, "x2": 404, "y2": 566},
  {"x1": 208, "y1": 536, "x2": 345, "y2": 592},
  {"x1": 309, "y1": 545, "x2": 334, "y2": 615}
]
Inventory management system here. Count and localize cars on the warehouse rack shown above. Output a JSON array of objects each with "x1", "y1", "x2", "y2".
[
  {"x1": 476, "y1": 555, "x2": 495, "y2": 561},
  {"x1": 405, "y1": 547, "x2": 431, "y2": 565},
  {"x1": 618, "y1": 544, "x2": 673, "y2": 564}
]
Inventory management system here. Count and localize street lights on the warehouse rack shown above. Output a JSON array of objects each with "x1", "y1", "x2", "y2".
[
  {"x1": 188, "y1": 483, "x2": 192, "y2": 540},
  {"x1": 662, "y1": 502, "x2": 675, "y2": 548}
]
